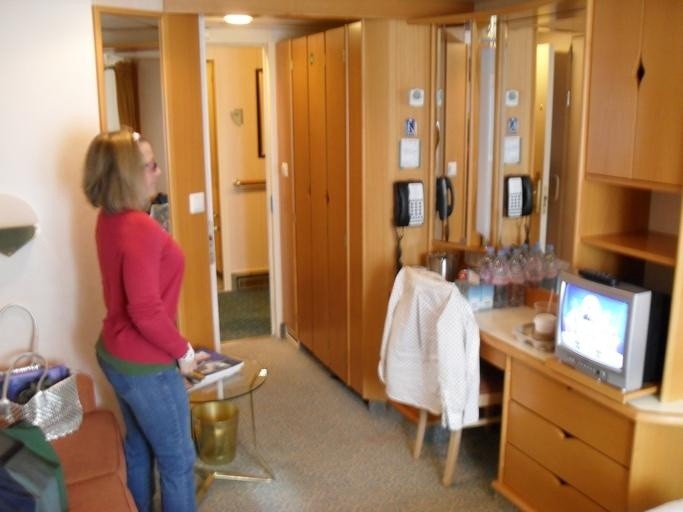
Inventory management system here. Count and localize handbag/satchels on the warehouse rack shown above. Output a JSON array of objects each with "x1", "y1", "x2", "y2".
[{"x1": 1, "y1": 362, "x2": 83, "y2": 442}]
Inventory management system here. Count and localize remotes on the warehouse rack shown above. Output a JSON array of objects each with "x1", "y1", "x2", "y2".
[{"x1": 578, "y1": 268, "x2": 619, "y2": 286}]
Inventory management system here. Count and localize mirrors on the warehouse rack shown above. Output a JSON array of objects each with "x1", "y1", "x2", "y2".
[
  {"x1": 90, "y1": 4, "x2": 173, "y2": 235},
  {"x1": 430, "y1": 1, "x2": 585, "y2": 258}
]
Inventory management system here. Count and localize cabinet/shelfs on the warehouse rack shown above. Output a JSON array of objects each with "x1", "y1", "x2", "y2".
[
  {"x1": 273, "y1": 17, "x2": 432, "y2": 407},
  {"x1": 475, "y1": 306, "x2": 683, "y2": 512},
  {"x1": 572, "y1": 0, "x2": 683, "y2": 402}
]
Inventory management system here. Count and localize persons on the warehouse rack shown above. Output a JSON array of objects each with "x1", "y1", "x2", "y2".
[{"x1": 81, "y1": 129, "x2": 200, "y2": 512}]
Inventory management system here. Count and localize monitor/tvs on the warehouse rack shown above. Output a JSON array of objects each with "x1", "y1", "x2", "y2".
[{"x1": 554, "y1": 269, "x2": 671, "y2": 394}]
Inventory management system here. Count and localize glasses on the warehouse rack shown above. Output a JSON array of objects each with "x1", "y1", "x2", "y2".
[{"x1": 145, "y1": 158, "x2": 157, "y2": 173}]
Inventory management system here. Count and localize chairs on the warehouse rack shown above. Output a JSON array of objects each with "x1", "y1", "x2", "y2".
[{"x1": 377, "y1": 264, "x2": 503, "y2": 489}]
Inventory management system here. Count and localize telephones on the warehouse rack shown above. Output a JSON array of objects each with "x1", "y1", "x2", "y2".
[
  {"x1": 437, "y1": 176, "x2": 454, "y2": 219},
  {"x1": 393, "y1": 180, "x2": 424, "y2": 226},
  {"x1": 504, "y1": 175, "x2": 532, "y2": 217}
]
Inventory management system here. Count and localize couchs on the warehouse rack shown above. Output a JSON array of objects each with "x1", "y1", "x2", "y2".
[{"x1": 49, "y1": 372, "x2": 140, "y2": 512}]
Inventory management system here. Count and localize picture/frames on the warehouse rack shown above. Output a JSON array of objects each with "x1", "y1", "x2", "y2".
[{"x1": 256, "y1": 68, "x2": 266, "y2": 158}]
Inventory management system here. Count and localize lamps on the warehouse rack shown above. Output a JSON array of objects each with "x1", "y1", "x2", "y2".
[{"x1": 0, "y1": 193, "x2": 39, "y2": 230}]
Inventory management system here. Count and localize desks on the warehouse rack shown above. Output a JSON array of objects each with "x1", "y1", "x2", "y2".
[{"x1": 187, "y1": 353, "x2": 280, "y2": 509}]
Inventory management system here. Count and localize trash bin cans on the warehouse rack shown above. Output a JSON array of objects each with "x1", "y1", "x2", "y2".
[{"x1": 190, "y1": 399, "x2": 238, "y2": 465}]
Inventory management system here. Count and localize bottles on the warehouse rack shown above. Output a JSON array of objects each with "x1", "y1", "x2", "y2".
[{"x1": 480, "y1": 240, "x2": 557, "y2": 309}]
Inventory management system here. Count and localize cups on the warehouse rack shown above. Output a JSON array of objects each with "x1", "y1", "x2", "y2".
[{"x1": 534, "y1": 302, "x2": 558, "y2": 342}]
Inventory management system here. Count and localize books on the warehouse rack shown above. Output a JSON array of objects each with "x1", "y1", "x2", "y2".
[{"x1": 179, "y1": 344, "x2": 245, "y2": 393}]
